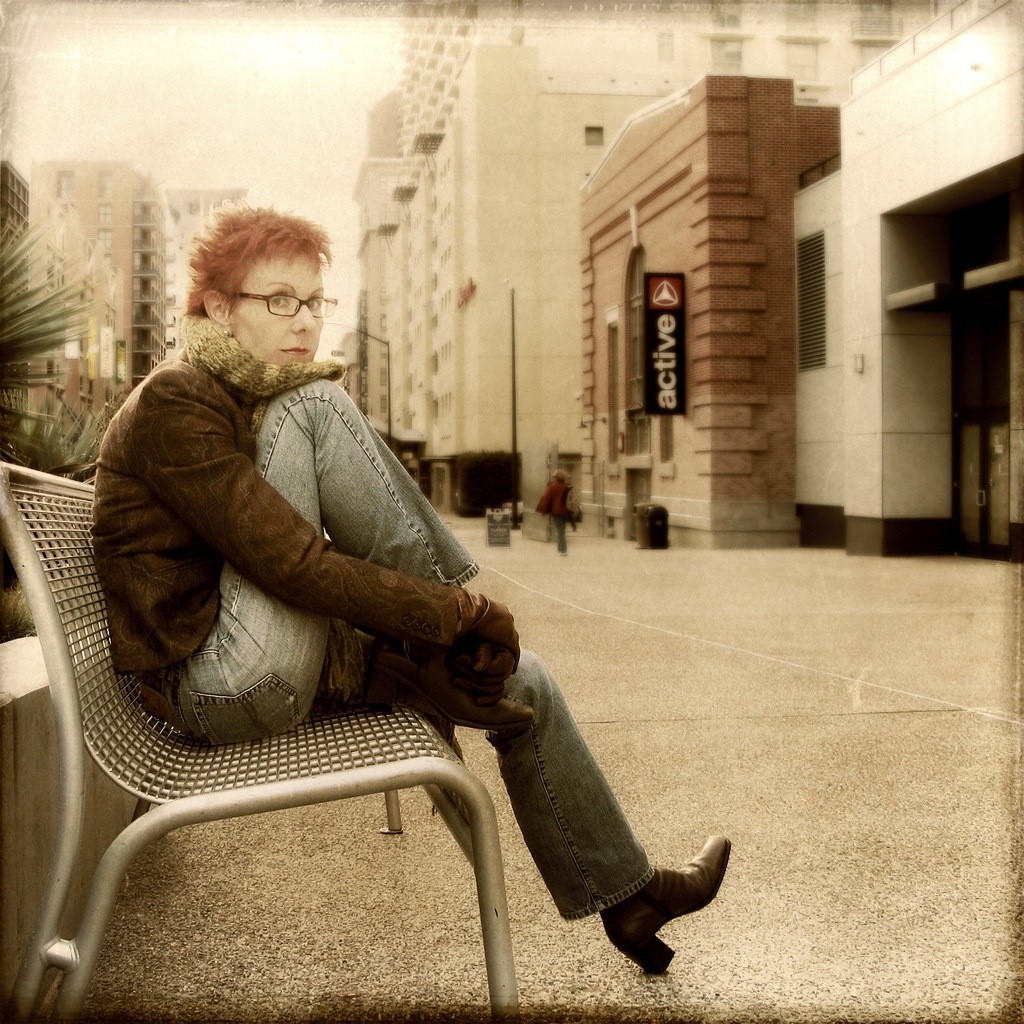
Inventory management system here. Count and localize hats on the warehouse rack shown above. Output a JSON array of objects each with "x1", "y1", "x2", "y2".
[{"x1": 552, "y1": 470, "x2": 570, "y2": 480}]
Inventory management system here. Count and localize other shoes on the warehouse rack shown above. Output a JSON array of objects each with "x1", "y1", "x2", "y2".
[{"x1": 557, "y1": 549, "x2": 567, "y2": 556}]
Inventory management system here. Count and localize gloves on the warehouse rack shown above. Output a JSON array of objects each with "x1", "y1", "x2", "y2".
[
  {"x1": 456, "y1": 587, "x2": 522, "y2": 675},
  {"x1": 452, "y1": 642, "x2": 515, "y2": 706}
]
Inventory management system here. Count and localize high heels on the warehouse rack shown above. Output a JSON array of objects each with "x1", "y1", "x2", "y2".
[
  {"x1": 365, "y1": 632, "x2": 535, "y2": 730},
  {"x1": 599, "y1": 837, "x2": 731, "y2": 976}
]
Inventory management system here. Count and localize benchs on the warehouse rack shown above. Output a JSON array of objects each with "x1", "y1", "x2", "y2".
[{"x1": 0, "y1": 461, "x2": 520, "y2": 1024}]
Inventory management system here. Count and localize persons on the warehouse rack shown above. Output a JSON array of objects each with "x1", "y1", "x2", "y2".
[
  {"x1": 538, "y1": 470, "x2": 577, "y2": 557},
  {"x1": 87, "y1": 207, "x2": 732, "y2": 974}
]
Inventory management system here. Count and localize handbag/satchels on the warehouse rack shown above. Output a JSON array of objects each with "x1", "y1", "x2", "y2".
[
  {"x1": 565, "y1": 485, "x2": 583, "y2": 510},
  {"x1": 568, "y1": 506, "x2": 583, "y2": 523}
]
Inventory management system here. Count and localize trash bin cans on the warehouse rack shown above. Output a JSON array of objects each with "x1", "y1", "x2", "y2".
[{"x1": 634, "y1": 502, "x2": 669, "y2": 549}]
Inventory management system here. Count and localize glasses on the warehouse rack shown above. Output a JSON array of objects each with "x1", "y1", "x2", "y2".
[{"x1": 238, "y1": 291, "x2": 338, "y2": 318}]
